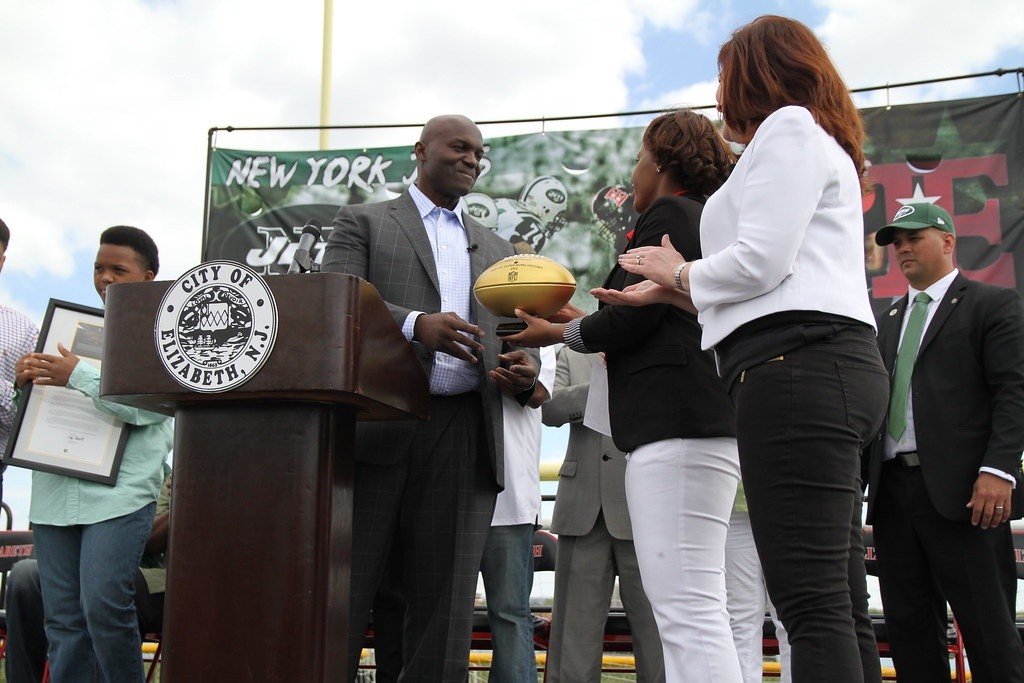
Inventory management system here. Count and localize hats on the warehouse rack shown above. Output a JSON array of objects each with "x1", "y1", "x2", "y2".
[{"x1": 875, "y1": 202, "x2": 956, "y2": 246}]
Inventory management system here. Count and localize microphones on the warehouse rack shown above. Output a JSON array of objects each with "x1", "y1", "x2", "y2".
[{"x1": 287, "y1": 218, "x2": 323, "y2": 274}]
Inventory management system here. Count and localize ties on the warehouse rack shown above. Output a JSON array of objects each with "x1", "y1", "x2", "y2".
[{"x1": 886, "y1": 292, "x2": 932, "y2": 443}]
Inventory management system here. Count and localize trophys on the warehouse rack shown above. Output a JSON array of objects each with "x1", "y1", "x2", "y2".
[{"x1": 472, "y1": 254, "x2": 577, "y2": 337}]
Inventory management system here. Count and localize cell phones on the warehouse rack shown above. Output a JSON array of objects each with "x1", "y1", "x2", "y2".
[{"x1": 496, "y1": 322, "x2": 528, "y2": 336}]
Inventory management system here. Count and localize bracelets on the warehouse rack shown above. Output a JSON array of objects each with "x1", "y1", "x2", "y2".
[{"x1": 674, "y1": 262, "x2": 689, "y2": 291}]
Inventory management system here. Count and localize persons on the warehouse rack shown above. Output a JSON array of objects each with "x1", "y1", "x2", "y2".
[
  {"x1": 590, "y1": 12, "x2": 894, "y2": 683},
  {"x1": 0, "y1": 217, "x2": 43, "y2": 515},
  {"x1": 4, "y1": 225, "x2": 170, "y2": 683},
  {"x1": 542, "y1": 205, "x2": 790, "y2": 683},
  {"x1": 875, "y1": 201, "x2": 1024, "y2": 683},
  {"x1": 320, "y1": 111, "x2": 556, "y2": 683},
  {"x1": 501, "y1": 107, "x2": 767, "y2": 683}
]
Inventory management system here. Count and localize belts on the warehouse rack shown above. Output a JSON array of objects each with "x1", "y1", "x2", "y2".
[
  {"x1": 899, "y1": 453, "x2": 920, "y2": 466},
  {"x1": 353, "y1": 392, "x2": 473, "y2": 420}
]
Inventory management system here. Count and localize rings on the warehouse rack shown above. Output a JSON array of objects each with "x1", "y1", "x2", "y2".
[
  {"x1": 996, "y1": 506, "x2": 1004, "y2": 510},
  {"x1": 636, "y1": 255, "x2": 642, "y2": 265}
]
